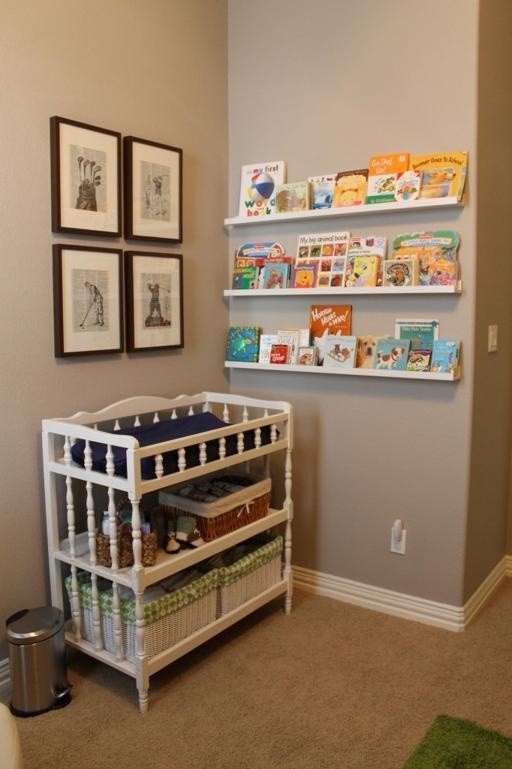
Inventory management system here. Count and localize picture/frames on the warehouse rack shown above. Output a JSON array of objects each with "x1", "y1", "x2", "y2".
[
  {"x1": 124, "y1": 248, "x2": 186, "y2": 355},
  {"x1": 50, "y1": 239, "x2": 126, "y2": 362},
  {"x1": 123, "y1": 134, "x2": 185, "y2": 244},
  {"x1": 47, "y1": 114, "x2": 124, "y2": 243}
]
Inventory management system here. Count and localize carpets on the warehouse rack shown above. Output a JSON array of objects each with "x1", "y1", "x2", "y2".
[{"x1": 403, "y1": 714, "x2": 512, "y2": 768}]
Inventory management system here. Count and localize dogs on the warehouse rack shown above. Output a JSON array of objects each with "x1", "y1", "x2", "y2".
[
  {"x1": 356, "y1": 335, "x2": 377, "y2": 368},
  {"x1": 377, "y1": 346, "x2": 404, "y2": 369}
]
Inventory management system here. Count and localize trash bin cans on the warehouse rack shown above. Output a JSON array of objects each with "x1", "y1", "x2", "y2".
[{"x1": 5, "y1": 606, "x2": 74, "y2": 718}]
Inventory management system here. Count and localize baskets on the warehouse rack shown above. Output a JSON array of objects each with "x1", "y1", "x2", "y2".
[
  {"x1": 215, "y1": 535, "x2": 288, "y2": 617},
  {"x1": 64, "y1": 559, "x2": 218, "y2": 661},
  {"x1": 155, "y1": 463, "x2": 275, "y2": 544}
]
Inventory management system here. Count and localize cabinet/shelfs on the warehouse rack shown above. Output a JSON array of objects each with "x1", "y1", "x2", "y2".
[
  {"x1": 38, "y1": 388, "x2": 297, "y2": 716},
  {"x1": 222, "y1": 195, "x2": 467, "y2": 384}
]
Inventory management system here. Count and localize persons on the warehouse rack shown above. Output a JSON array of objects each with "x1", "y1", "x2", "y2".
[
  {"x1": 85, "y1": 281, "x2": 104, "y2": 326},
  {"x1": 147, "y1": 284, "x2": 162, "y2": 319}
]
